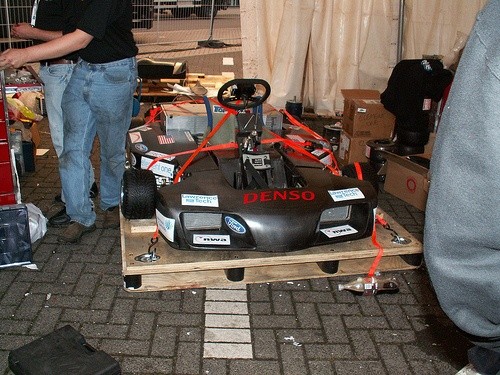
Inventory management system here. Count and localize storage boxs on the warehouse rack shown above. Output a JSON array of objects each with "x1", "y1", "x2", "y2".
[{"x1": 339, "y1": 90, "x2": 436, "y2": 212}]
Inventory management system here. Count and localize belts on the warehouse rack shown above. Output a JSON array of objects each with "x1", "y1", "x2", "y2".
[{"x1": 40, "y1": 59, "x2": 71, "y2": 66}]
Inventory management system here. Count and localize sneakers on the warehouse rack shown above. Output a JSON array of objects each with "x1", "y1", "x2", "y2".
[
  {"x1": 103, "y1": 206, "x2": 119, "y2": 228},
  {"x1": 63, "y1": 221, "x2": 96, "y2": 241}
]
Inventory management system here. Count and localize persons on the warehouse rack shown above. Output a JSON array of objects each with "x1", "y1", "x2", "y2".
[
  {"x1": 0, "y1": 0, "x2": 139, "y2": 244},
  {"x1": 423, "y1": 0, "x2": 500, "y2": 375}
]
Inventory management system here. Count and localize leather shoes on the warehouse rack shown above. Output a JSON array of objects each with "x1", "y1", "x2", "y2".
[
  {"x1": 55, "y1": 182, "x2": 98, "y2": 202},
  {"x1": 50, "y1": 214, "x2": 70, "y2": 226}
]
